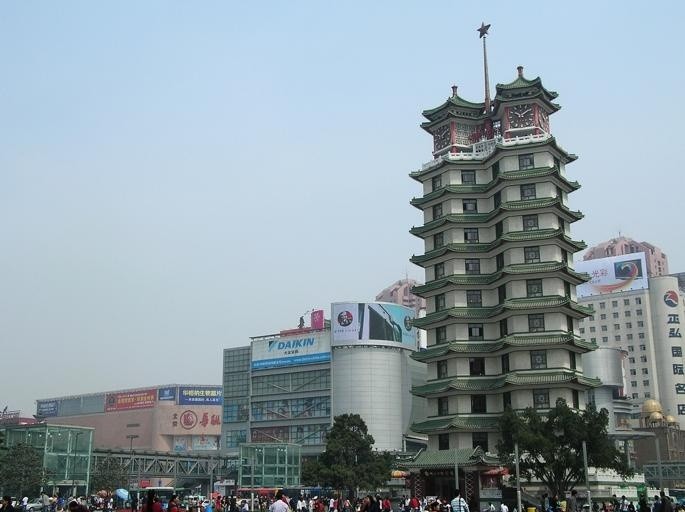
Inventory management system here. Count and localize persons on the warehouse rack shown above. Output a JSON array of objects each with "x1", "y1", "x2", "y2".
[
  {"x1": 338, "y1": 312, "x2": 352, "y2": 325},
  {"x1": 1, "y1": 487, "x2": 685, "y2": 511}
]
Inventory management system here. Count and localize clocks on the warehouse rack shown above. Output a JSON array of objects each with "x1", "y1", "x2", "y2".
[
  {"x1": 509, "y1": 104, "x2": 534, "y2": 128},
  {"x1": 538, "y1": 107, "x2": 549, "y2": 131},
  {"x1": 434, "y1": 125, "x2": 450, "y2": 150}
]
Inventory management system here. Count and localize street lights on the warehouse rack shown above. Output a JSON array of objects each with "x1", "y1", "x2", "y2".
[
  {"x1": 639, "y1": 397, "x2": 679, "y2": 494},
  {"x1": 125, "y1": 433, "x2": 140, "y2": 491},
  {"x1": 69, "y1": 431, "x2": 85, "y2": 496}
]
